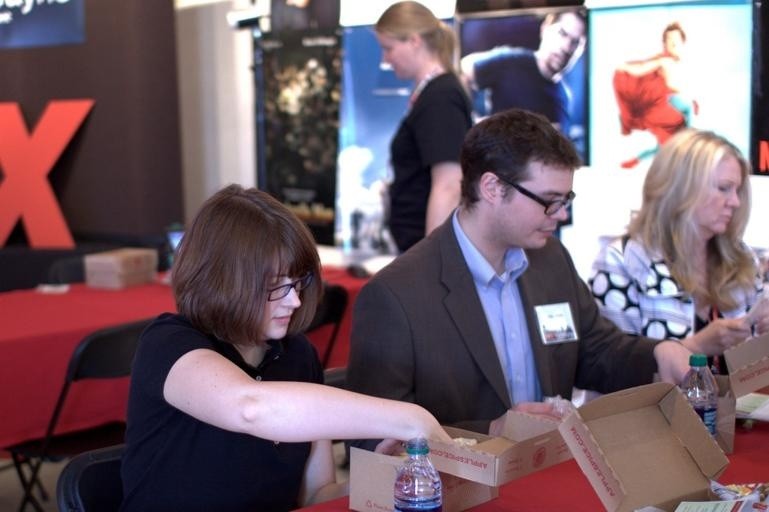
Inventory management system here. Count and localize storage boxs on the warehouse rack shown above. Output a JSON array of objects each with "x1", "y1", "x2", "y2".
[
  {"x1": 349, "y1": 411, "x2": 574, "y2": 512},
  {"x1": 652, "y1": 331, "x2": 769, "y2": 455},
  {"x1": 557, "y1": 382, "x2": 769, "y2": 512}
]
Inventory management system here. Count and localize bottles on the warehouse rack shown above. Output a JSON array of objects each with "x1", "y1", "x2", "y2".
[
  {"x1": 394, "y1": 438, "x2": 444, "y2": 512},
  {"x1": 682, "y1": 352, "x2": 717, "y2": 443}
]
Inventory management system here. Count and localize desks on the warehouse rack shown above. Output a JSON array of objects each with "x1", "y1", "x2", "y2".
[
  {"x1": 0, "y1": 266, "x2": 369, "y2": 445},
  {"x1": 287, "y1": 385, "x2": 769, "y2": 512}
]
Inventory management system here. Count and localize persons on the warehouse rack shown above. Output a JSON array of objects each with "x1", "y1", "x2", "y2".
[
  {"x1": 122, "y1": 184, "x2": 477, "y2": 512},
  {"x1": 372, "y1": 1, "x2": 473, "y2": 260},
  {"x1": 574, "y1": 131, "x2": 769, "y2": 407},
  {"x1": 459, "y1": 5, "x2": 591, "y2": 145},
  {"x1": 610, "y1": 22, "x2": 696, "y2": 167},
  {"x1": 265, "y1": 46, "x2": 341, "y2": 214},
  {"x1": 344, "y1": 107, "x2": 718, "y2": 473}
]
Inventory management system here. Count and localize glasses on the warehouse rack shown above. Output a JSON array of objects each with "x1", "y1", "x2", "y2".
[
  {"x1": 262, "y1": 269, "x2": 314, "y2": 301},
  {"x1": 499, "y1": 174, "x2": 575, "y2": 215}
]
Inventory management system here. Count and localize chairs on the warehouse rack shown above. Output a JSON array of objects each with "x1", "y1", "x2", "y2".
[
  {"x1": 0, "y1": 316, "x2": 159, "y2": 512},
  {"x1": 302, "y1": 282, "x2": 351, "y2": 371}
]
